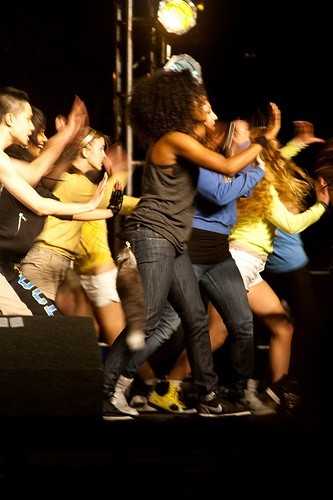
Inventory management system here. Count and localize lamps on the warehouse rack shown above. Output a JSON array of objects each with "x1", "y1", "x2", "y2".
[{"x1": 151, "y1": 0, "x2": 205, "y2": 39}]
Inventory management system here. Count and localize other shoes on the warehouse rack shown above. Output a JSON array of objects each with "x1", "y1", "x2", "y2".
[
  {"x1": 265, "y1": 384, "x2": 299, "y2": 416},
  {"x1": 127, "y1": 394, "x2": 157, "y2": 412},
  {"x1": 103, "y1": 399, "x2": 125, "y2": 416}
]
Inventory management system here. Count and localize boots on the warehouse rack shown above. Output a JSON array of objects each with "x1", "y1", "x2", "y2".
[
  {"x1": 110, "y1": 375, "x2": 139, "y2": 416},
  {"x1": 235, "y1": 379, "x2": 274, "y2": 414},
  {"x1": 147, "y1": 378, "x2": 197, "y2": 413}
]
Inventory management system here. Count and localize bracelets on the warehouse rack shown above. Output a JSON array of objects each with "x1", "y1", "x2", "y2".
[{"x1": 255, "y1": 135, "x2": 267, "y2": 148}]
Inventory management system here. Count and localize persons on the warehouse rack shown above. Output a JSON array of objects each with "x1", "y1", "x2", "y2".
[{"x1": 0, "y1": 69, "x2": 333, "y2": 420}]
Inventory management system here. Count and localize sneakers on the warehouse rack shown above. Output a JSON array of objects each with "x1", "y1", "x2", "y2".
[{"x1": 199, "y1": 388, "x2": 251, "y2": 417}]
